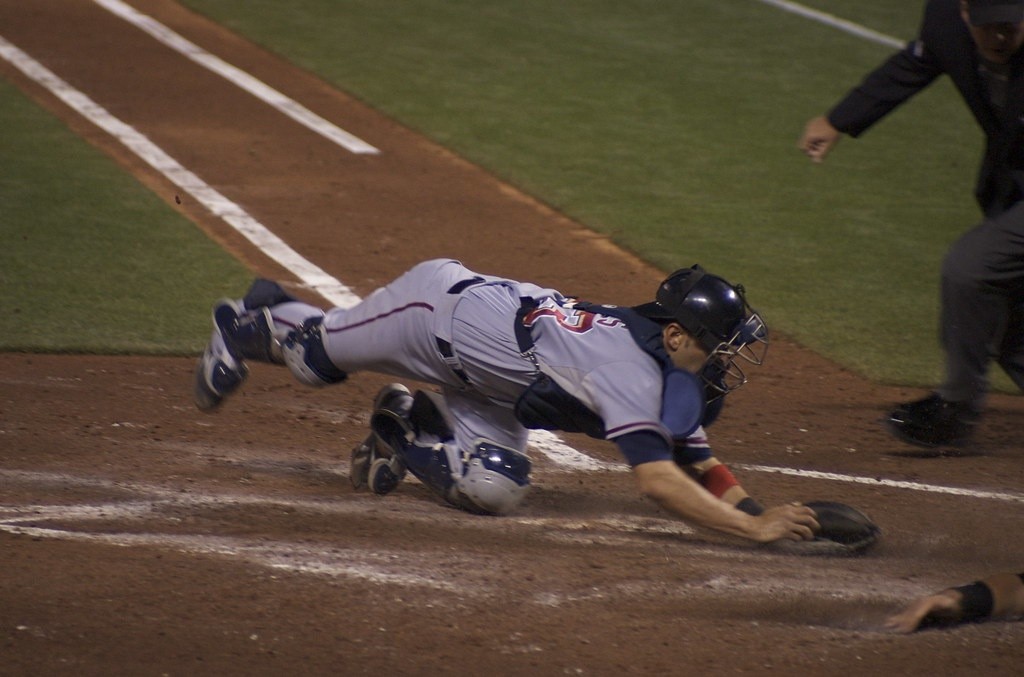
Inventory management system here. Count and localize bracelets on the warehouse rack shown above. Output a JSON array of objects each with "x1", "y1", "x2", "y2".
[{"x1": 949, "y1": 581, "x2": 994, "y2": 623}]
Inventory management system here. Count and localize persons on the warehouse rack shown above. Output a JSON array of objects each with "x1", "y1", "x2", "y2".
[
  {"x1": 196, "y1": 256, "x2": 822, "y2": 548},
  {"x1": 798, "y1": 0, "x2": 1024, "y2": 449},
  {"x1": 881, "y1": 572, "x2": 1024, "y2": 634}
]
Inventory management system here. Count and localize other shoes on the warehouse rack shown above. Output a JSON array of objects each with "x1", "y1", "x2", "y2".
[{"x1": 887, "y1": 395, "x2": 973, "y2": 456}]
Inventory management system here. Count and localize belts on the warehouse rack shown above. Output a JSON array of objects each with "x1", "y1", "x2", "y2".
[{"x1": 435, "y1": 275, "x2": 486, "y2": 388}]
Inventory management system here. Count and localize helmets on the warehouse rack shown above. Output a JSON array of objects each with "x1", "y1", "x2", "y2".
[{"x1": 656, "y1": 265, "x2": 770, "y2": 408}]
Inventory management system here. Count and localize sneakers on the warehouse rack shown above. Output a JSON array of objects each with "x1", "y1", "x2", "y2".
[
  {"x1": 349, "y1": 382, "x2": 414, "y2": 492},
  {"x1": 192, "y1": 292, "x2": 250, "y2": 413}
]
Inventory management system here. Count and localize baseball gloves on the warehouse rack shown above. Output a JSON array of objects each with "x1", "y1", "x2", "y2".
[{"x1": 779, "y1": 499, "x2": 881, "y2": 556}]
